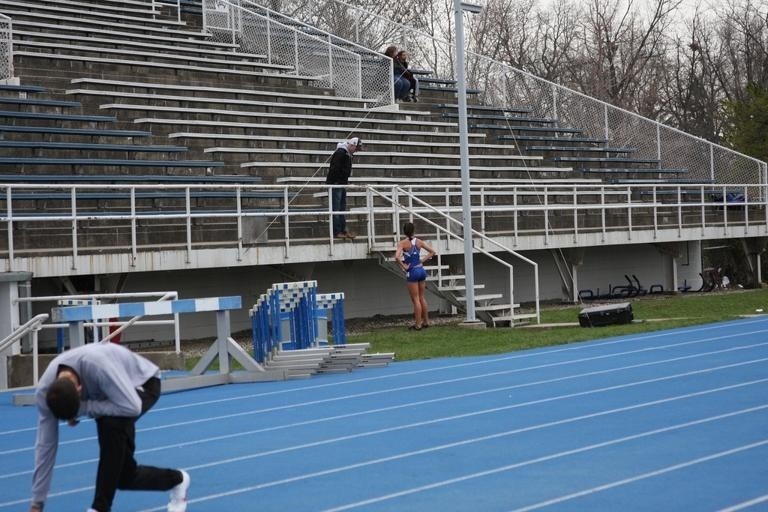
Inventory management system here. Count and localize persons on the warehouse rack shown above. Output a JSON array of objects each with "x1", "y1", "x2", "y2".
[
  {"x1": 325, "y1": 137, "x2": 363, "y2": 239},
  {"x1": 398, "y1": 50, "x2": 418, "y2": 103},
  {"x1": 28, "y1": 339, "x2": 191, "y2": 511},
  {"x1": 394, "y1": 223, "x2": 437, "y2": 332},
  {"x1": 385, "y1": 46, "x2": 411, "y2": 102}
]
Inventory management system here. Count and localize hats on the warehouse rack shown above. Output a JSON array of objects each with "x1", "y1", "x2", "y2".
[{"x1": 350, "y1": 137, "x2": 364, "y2": 151}]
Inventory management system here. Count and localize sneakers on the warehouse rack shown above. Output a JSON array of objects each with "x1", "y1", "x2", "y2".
[
  {"x1": 167, "y1": 469, "x2": 190, "y2": 512},
  {"x1": 336, "y1": 232, "x2": 355, "y2": 238},
  {"x1": 408, "y1": 324, "x2": 428, "y2": 331}
]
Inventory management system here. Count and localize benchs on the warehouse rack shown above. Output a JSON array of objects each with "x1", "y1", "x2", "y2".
[{"x1": 0, "y1": 0, "x2": 738, "y2": 227}]
